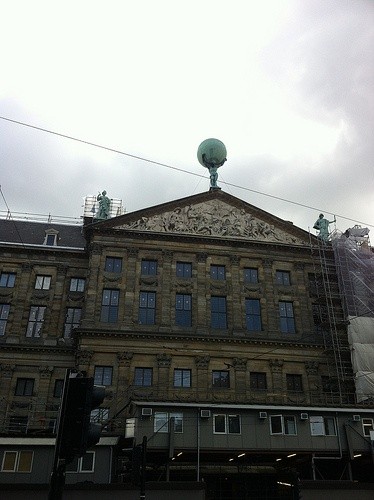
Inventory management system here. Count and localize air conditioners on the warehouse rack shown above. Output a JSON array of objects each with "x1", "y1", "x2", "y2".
[
  {"x1": 141, "y1": 407, "x2": 153, "y2": 416},
  {"x1": 200, "y1": 410, "x2": 211, "y2": 417},
  {"x1": 260, "y1": 411, "x2": 268, "y2": 419},
  {"x1": 300, "y1": 412, "x2": 309, "y2": 420}
]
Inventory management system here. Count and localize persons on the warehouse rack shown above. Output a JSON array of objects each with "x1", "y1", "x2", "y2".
[
  {"x1": 94, "y1": 191, "x2": 111, "y2": 220},
  {"x1": 200, "y1": 154, "x2": 227, "y2": 187},
  {"x1": 314, "y1": 213, "x2": 336, "y2": 239}
]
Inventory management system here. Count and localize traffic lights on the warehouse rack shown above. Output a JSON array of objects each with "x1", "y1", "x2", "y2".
[{"x1": 56, "y1": 368, "x2": 107, "y2": 463}]
